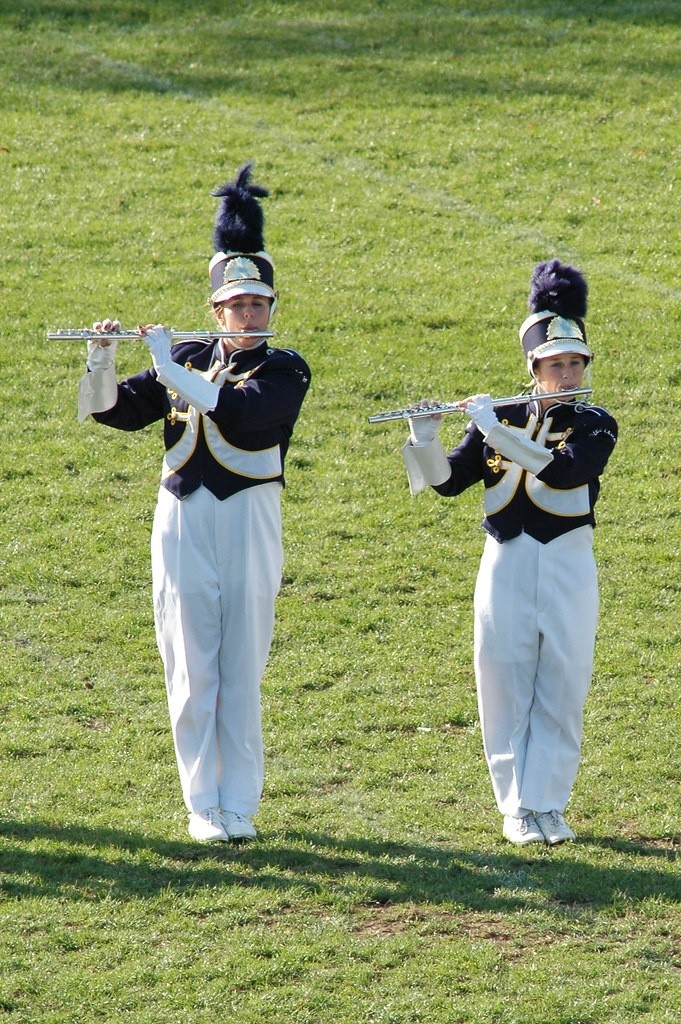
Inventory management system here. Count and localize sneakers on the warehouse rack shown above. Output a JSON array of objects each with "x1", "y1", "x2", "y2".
[
  {"x1": 187, "y1": 807, "x2": 229, "y2": 843},
  {"x1": 218, "y1": 810, "x2": 256, "y2": 840},
  {"x1": 503, "y1": 813, "x2": 545, "y2": 846},
  {"x1": 534, "y1": 809, "x2": 575, "y2": 845}
]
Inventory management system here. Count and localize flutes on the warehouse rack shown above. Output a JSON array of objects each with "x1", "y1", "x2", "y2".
[
  {"x1": 46, "y1": 328, "x2": 274, "y2": 341},
  {"x1": 367, "y1": 387, "x2": 593, "y2": 424}
]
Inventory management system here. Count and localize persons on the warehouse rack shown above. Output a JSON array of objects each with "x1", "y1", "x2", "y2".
[
  {"x1": 410, "y1": 309, "x2": 618, "y2": 847},
  {"x1": 73, "y1": 251, "x2": 311, "y2": 846}
]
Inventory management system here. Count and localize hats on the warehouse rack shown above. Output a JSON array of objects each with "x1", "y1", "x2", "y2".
[
  {"x1": 518, "y1": 259, "x2": 592, "y2": 379},
  {"x1": 207, "y1": 162, "x2": 275, "y2": 302}
]
